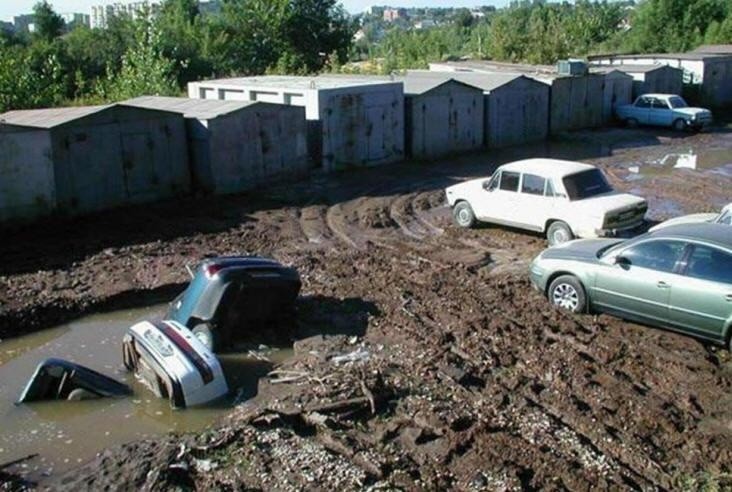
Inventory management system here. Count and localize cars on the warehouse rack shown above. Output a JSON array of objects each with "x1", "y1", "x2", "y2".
[
  {"x1": 620, "y1": 90, "x2": 712, "y2": 135},
  {"x1": 537, "y1": 205, "x2": 728, "y2": 350},
  {"x1": 121, "y1": 319, "x2": 230, "y2": 406},
  {"x1": 443, "y1": 157, "x2": 649, "y2": 244},
  {"x1": 18, "y1": 359, "x2": 133, "y2": 402},
  {"x1": 162, "y1": 255, "x2": 302, "y2": 354}
]
